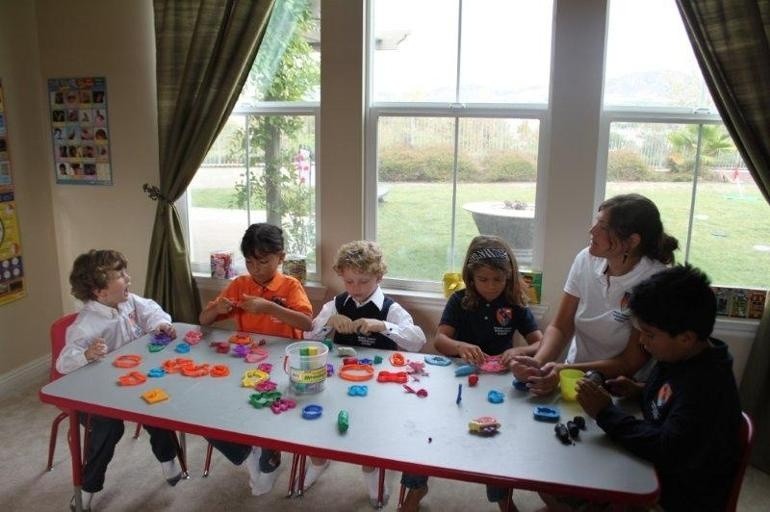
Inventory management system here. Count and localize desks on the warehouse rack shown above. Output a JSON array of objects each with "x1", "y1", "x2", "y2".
[
  {"x1": 461, "y1": 199, "x2": 537, "y2": 273},
  {"x1": 36, "y1": 321, "x2": 662, "y2": 512}
]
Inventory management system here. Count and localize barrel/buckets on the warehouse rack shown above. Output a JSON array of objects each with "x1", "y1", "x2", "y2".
[
  {"x1": 282, "y1": 255, "x2": 308, "y2": 288},
  {"x1": 282, "y1": 340, "x2": 329, "y2": 398}
]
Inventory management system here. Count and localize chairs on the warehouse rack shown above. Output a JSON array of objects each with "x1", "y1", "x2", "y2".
[
  {"x1": 721, "y1": 406, "x2": 758, "y2": 511},
  {"x1": 43, "y1": 311, "x2": 194, "y2": 483}
]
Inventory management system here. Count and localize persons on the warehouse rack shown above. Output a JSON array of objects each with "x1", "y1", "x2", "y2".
[
  {"x1": 199, "y1": 224, "x2": 314, "y2": 495},
  {"x1": 513, "y1": 194, "x2": 677, "y2": 396},
  {"x1": 399, "y1": 236, "x2": 543, "y2": 512},
  {"x1": 56, "y1": 249, "x2": 182, "y2": 512},
  {"x1": 539, "y1": 263, "x2": 740, "y2": 512},
  {"x1": 295, "y1": 240, "x2": 426, "y2": 508}
]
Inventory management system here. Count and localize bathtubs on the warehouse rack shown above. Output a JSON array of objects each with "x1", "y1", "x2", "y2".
[{"x1": 462, "y1": 200, "x2": 537, "y2": 251}]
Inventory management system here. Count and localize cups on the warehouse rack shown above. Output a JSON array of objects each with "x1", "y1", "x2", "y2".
[
  {"x1": 281, "y1": 254, "x2": 307, "y2": 285},
  {"x1": 559, "y1": 368, "x2": 588, "y2": 403}
]
